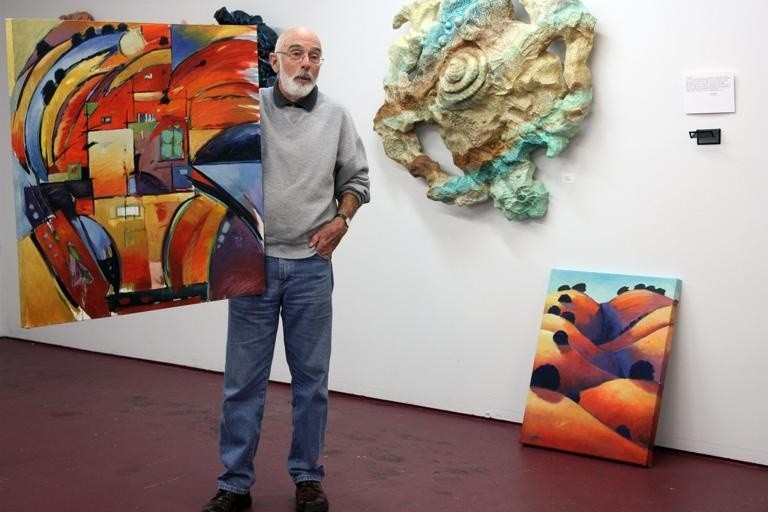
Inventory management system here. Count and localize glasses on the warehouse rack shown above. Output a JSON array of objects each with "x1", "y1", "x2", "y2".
[{"x1": 277, "y1": 50, "x2": 324, "y2": 65}]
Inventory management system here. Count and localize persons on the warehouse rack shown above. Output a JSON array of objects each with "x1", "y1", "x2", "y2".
[{"x1": 200, "y1": 27, "x2": 369, "y2": 512}]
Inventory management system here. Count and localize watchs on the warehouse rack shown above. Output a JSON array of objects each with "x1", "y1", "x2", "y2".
[{"x1": 334, "y1": 213, "x2": 351, "y2": 229}]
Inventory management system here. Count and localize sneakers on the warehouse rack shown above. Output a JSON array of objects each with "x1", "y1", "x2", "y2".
[
  {"x1": 204, "y1": 490, "x2": 251, "y2": 512},
  {"x1": 296, "y1": 481, "x2": 328, "y2": 511}
]
